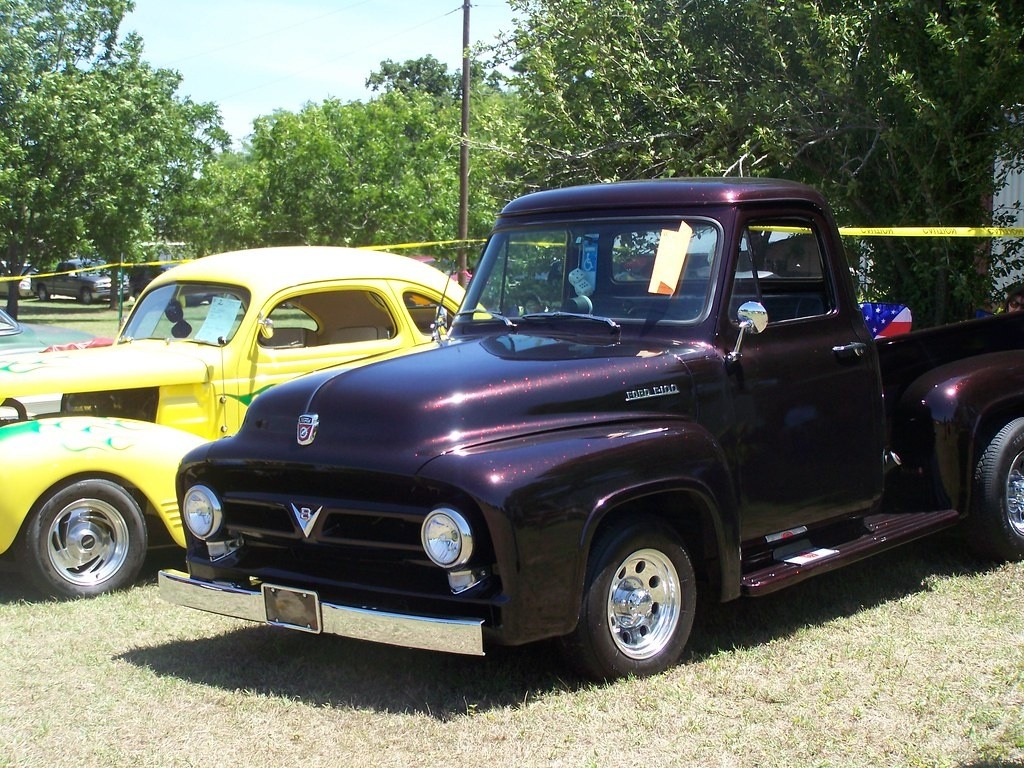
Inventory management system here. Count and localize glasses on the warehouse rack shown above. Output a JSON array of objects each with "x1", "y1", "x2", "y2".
[
  {"x1": 165, "y1": 298, "x2": 192, "y2": 339},
  {"x1": 1010, "y1": 300, "x2": 1024, "y2": 309}
]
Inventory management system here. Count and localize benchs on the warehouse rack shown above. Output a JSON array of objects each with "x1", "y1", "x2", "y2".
[{"x1": 600, "y1": 294, "x2": 826, "y2": 323}]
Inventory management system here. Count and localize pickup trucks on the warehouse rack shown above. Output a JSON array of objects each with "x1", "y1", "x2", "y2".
[
  {"x1": 30, "y1": 258, "x2": 130, "y2": 305},
  {"x1": 157, "y1": 177, "x2": 1023, "y2": 684}
]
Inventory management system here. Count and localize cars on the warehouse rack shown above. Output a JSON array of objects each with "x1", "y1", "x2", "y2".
[
  {"x1": 0, "y1": 260, "x2": 39, "y2": 299},
  {"x1": 0, "y1": 244, "x2": 493, "y2": 600},
  {"x1": 0, "y1": 306, "x2": 118, "y2": 358}
]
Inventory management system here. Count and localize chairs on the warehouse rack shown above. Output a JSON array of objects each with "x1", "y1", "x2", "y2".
[
  {"x1": 329, "y1": 326, "x2": 388, "y2": 345},
  {"x1": 258, "y1": 328, "x2": 318, "y2": 347}
]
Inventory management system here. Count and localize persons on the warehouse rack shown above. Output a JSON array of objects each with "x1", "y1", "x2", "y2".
[{"x1": 1006, "y1": 283, "x2": 1024, "y2": 312}]
